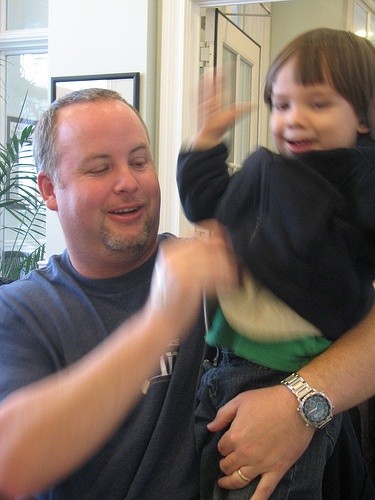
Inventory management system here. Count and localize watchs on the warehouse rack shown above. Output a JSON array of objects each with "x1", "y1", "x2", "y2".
[{"x1": 282, "y1": 372, "x2": 336, "y2": 429}]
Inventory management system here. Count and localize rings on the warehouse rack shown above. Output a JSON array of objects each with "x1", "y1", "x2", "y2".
[{"x1": 238, "y1": 469, "x2": 251, "y2": 483}]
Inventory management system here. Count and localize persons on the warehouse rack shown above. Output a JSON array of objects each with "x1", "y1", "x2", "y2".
[
  {"x1": 173, "y1": 29, "x2": 374, "y2": 499},
  {"x1": 0, "y1": 86, "x2": 375, "y2": 500}
]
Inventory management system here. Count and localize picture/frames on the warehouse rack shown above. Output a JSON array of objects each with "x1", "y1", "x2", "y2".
[
  {"x1": 51, "y1": 72, "x2": 139, "y2": 111},
  {"x1": 7, "y1": 116, "x2": 47, "y2": 210}
]
